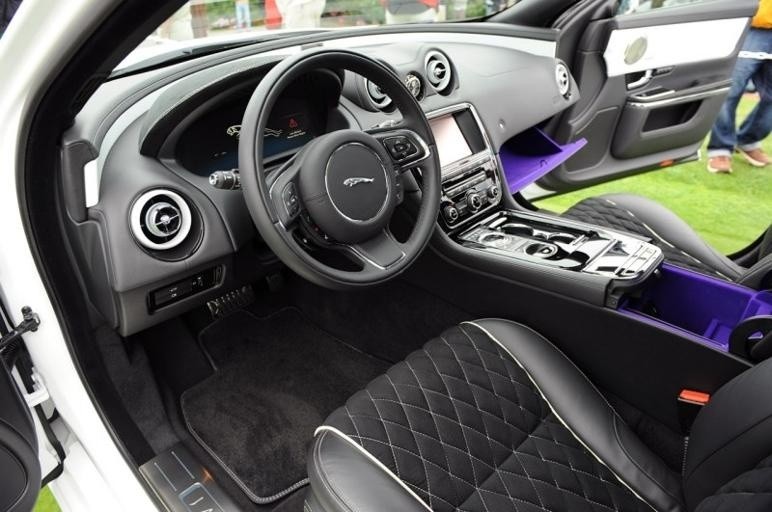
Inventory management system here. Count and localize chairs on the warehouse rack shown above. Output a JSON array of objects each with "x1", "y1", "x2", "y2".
[
  {"x1": 555, "y1": 191, "x2": 771, "y2": 291},
  {"x1": 298, "y1": 309, "x2": 772, "y2": 511}
]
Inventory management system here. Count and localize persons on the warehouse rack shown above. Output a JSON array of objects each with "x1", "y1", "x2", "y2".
[
  {"x1": 234, "y1": 0, "x2": 440, "y2": 33},
  {"x1": 706, "y1": 1, "x2": 771, "y2": 175}
]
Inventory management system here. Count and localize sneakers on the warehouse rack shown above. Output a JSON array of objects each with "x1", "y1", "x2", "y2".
[
  {"x1": 742, "y1": 149, "x2": 771, "y2": 167},
  {"x1": 708, "y1": 156, "x2": 733, "y2": 175}
]
